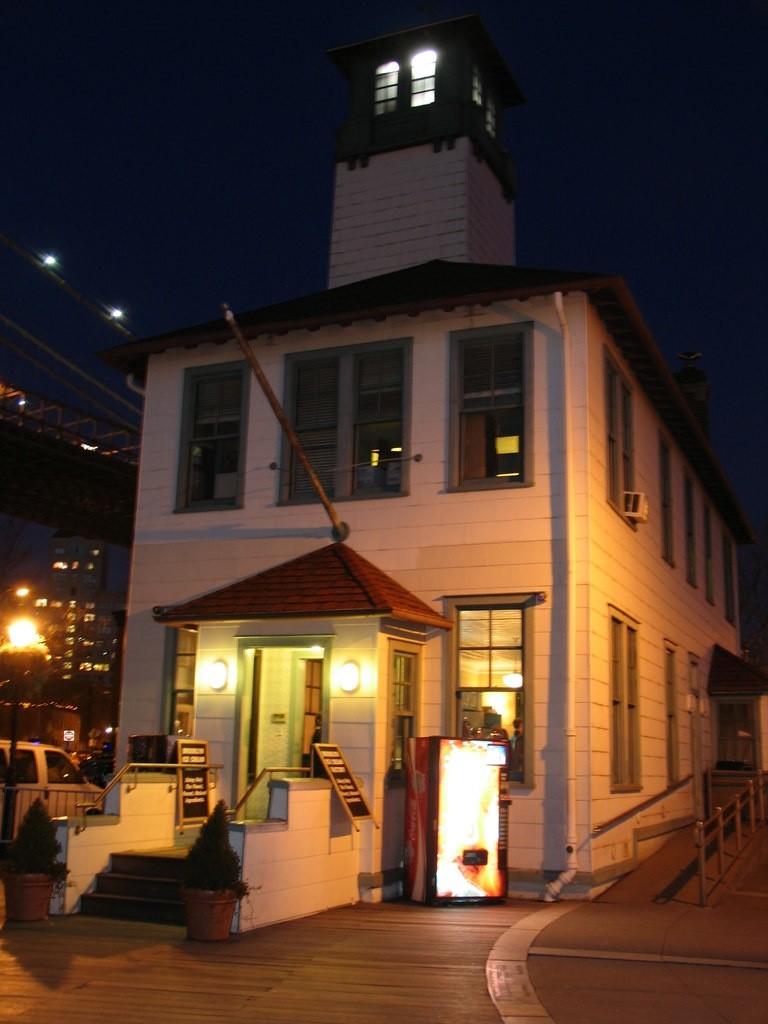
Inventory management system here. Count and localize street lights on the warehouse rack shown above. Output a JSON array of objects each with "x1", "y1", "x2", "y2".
[{"x1": 0, "y1": 619, "x2": 40, "y2": 847}]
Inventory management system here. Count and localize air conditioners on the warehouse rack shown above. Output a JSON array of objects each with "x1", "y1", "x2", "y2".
[{"x1": 624, "y1": 490, "x2": 650, "y2": 523}]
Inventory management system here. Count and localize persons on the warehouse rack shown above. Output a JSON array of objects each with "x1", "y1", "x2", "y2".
[
  {"x1": 463, "y1": 717, "x2": 523, "y2": 748},
  {"x1": 312, "y1": 712, "x2": 321, "y2": 743}
]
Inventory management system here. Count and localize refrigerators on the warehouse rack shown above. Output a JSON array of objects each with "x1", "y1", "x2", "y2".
[{"x1": 405, "y1": 734, "x2": 512, "y2": 905}]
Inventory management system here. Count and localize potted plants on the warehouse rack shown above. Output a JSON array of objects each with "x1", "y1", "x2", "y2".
[
  {"x1": 0, "y1": 799, "x2": 71, "y2": 922},
  {"x1": 180, "y1": 799, "x2": 249, "y2": 941}
]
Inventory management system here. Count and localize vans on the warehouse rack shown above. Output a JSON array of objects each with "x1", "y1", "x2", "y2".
[{"x1": 0, "y1": 739, "x2": 104, "y2": 841}]
